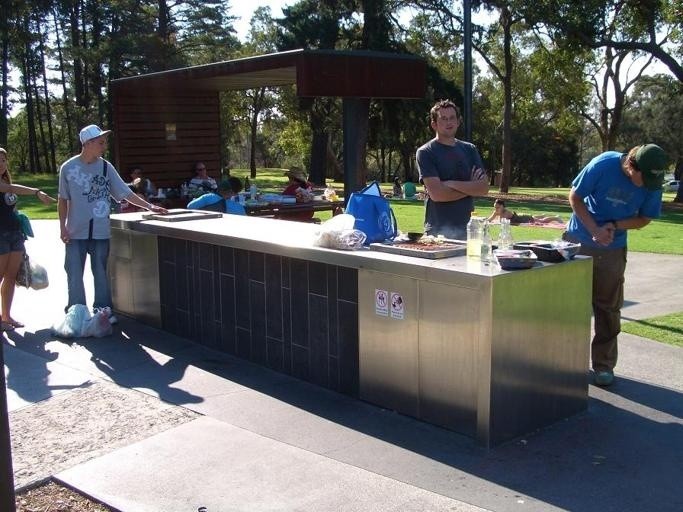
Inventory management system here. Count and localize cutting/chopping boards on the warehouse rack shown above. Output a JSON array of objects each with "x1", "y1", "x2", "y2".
[
  {"x1": 141, "y1": 209, "x2": 223, "y2": 222},
  {"x1": 369, "y1": 239, "x2": 496, "y2": 259}
]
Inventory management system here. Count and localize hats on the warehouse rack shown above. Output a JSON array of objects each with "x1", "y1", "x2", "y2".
[
  {"x1": 285, "y1": 166, "x2": 307, "y2": 182},
  {"x1": 79, "y1": 124, "x2": 111, "y2": 145},
  {"x1": 635, "y1": 143, "x2": 666, "y2": 190}
]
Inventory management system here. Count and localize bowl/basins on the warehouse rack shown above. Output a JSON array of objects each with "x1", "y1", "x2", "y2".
[
  {"x1": 407, "y1": 232, "x2": 423, "y2": 243},
  {"x1": 494, "y1": 241, "x2": 581, "y2": 270}
]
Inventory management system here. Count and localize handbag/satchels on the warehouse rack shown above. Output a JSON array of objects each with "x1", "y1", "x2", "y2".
[
  {"x1": 17, "y1": 255, "x2": 31, "y2": 287},
  {"x1": 345, "y1": 182, "x2": 397, "y2": 246}
]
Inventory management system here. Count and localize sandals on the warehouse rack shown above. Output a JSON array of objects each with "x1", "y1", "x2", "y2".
[
  {"x1": 0, "y1": 321, "x2": 14, "y2": 331},
  {"x1": 5, "y1": 319, "x2": 23, "y2": 327}
]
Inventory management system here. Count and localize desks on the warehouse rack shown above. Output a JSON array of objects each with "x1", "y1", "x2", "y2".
[{"x1": 244, "y1": 195, "x2": 346, "y2": 216}]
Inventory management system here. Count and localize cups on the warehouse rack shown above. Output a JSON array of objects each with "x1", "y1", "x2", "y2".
[
  {"x1": 158, "y1": 188, "x2": 163, "y2": 198},
  {"x1": 313, "y1": 196, "x2": 322, "y2": 200},
  {"x1": 239, "y1": 194, "x2": 245, "y2": 204},
  {"x1": 331, "y1": 195, "x2": 339, "y2": 202}
]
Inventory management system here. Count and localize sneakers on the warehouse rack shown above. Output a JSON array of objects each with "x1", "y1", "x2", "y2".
[
  {"x1": 594, "y1": 370, "x2": 614, "y2": 386},
  {"x1": 558, "y1": 216, "x2": 562, "y2": 223},
  {"x1": 93, "y1": 306, "x2": 117, "y2": 324}
]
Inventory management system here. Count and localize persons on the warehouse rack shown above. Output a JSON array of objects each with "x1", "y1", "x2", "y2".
[
  {"x1": 562, "y1": 144, "x2": 667, "y2": 385},
  {"x1": 186, "y1": 161, "x2": 245, "y2": 213},
  {"x1": 0, "y1": 147, "x2": 57, "y2": 330},
  {"x1": 272, "y1": 166, "x2": 314, "y2": 220},
  {"x1": 117, "y1": 185, "x2": 147, "y2": 212},
  {"x1": 415, "y1": 99, "x2": 490, "y2": 240},
  {"x1": 419, "y1": 185, "x2": 430, "y2": 199},
  {"x1": 58, "y1": 124, "x2": 168, "y2": 318},
  {"x1": 391, "y1": 177, "x2": 403, "y2": 198},
  {"x1": 131, "y1": 169, "x2": 155, "y2": 196},
  {"x1": 402, "y1": 177, "x2": 419, "y2": 200},
  {"x1": 486, "y1": 199, "x2": 564, "y2": 226}
]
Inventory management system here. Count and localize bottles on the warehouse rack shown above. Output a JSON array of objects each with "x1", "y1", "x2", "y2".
[
  {"x1": 480, "y1": 219, "x2": 490, "y2": 264},
  {"x1": 504, "y1": 219, "x2": 513, "y2": 249},
  {"x1": 250, "y1": 184, "x2": 257, "y2": 200},
  {"x1": 231, "y1": 196, "x2": 236, "y2": 202},
  {"x1": 467, "y1": 211, "x2": 482, "y2": 257},
  {"x1": 183, "y1": 181, "x2": 188, "y2": 195},
  {"x1": 498, "y1": 218, "x2": 506, "y2": 249}
]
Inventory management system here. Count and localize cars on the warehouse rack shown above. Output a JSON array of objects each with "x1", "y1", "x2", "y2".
[{"x1": 661, "y1": 180, "x2": 681, "y2": 192}]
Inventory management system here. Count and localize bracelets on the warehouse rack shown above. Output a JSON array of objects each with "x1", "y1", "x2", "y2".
[
  {"x1": 35, "y1": 188, "x2": 42, "y2": 195},
  {"x1": 610, "y1": 220, "x2": 618, "y2": 230},
  {"x1": 147, "y1": 203, "x2": 151, "y2": 210}
]
